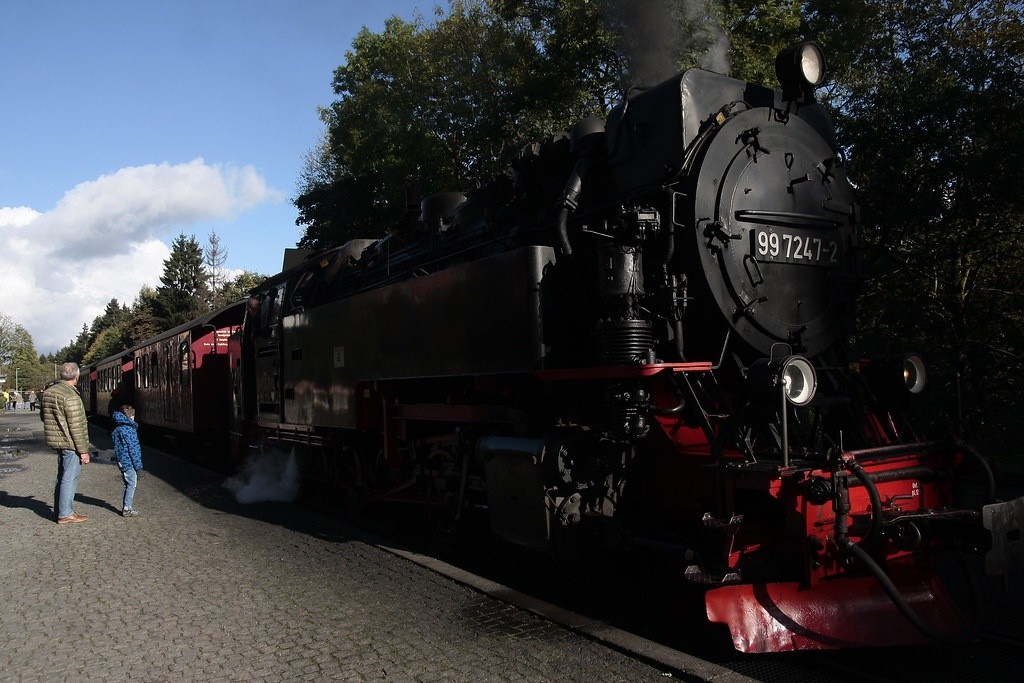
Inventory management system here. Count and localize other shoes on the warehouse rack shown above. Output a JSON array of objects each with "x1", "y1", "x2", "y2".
[
  {"x1": 58, "y1": 513, "x2": 88, "y2": 524},
  {"x1": 123, "y1": 508, "x2": 140, "y2": 517}
]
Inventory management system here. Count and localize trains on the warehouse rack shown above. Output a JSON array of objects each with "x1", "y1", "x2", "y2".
[{"x1": 54, "y1": 43, "x2": 1024, "y2": 654}]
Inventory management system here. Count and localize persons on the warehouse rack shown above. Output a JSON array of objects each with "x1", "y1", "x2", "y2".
[
  {"x1": 111, "y1": 403, "x2": 145, "y2": 522},
  {"x1": 3, "y1": 389, "x2": 37, "y2": 411},
  {"x1": 41, "y1": 362, "x2": 90, "y2": 528}
]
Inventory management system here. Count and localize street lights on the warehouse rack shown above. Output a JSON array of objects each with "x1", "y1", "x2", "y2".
[{"x1": 16, "y1": 368, "x2": 20, "y2": 390}]
